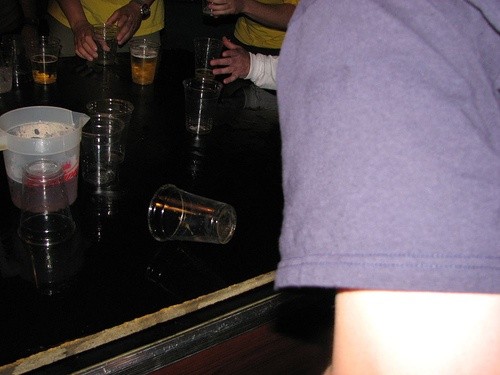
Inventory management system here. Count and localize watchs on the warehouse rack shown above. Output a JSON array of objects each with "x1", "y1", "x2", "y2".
[{"x1": 130, "y1": 0, "x2": 151, "y2": 20}]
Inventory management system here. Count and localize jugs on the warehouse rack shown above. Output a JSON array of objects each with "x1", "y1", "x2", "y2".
[{"x1": 0, "y1": 106, "x2": 90, "y2": 214}]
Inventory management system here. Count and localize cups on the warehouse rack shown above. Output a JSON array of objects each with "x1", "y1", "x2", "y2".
[
  {"x1": 16, "y1": 159, "x2": 76, "y2": 245},
  {"x1": 183, "y1": 79, "x2": 222, "y2": 130},
  {"x1": 91, "y1": 24, "x2": 120, "y2": 65},
  {"x1": 80, "y1": 116, "x2": 125, "y2": 186},
  {"x1": 2, "y1": 36, "x2": 23, "y2": 70},
  {"x1": 90, "y1": 189, "x2": 120, "y2": 239},
  {"x1": 202, "y1": 1, "x2": 219, "y2": 20},
  {"x1": 192, "y1": 38, "x2": 224, "y2": 82},
  {"x1": 83, "y1": 100, "x2": 134, "y2": 150},
  {"x1": 17, "y1": 234, "x2": 71, "y2": 294},
  {"x1": 128, "y1": 38, "x2": 159, "y2": 85},
  {"x1": 25, "y1": 37, "x2": 60, "y2": 84},
  {"x1": 147, "y1": 184, "x2": 237, "y2": 243}
]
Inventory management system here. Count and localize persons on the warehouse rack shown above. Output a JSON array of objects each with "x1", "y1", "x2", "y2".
[
  {"x1": 1, "y1": 0, "x2": 295, "y2": 93},
  {"x1": 274, "y1": 0, "x2": 500, "y2": 375}
]
